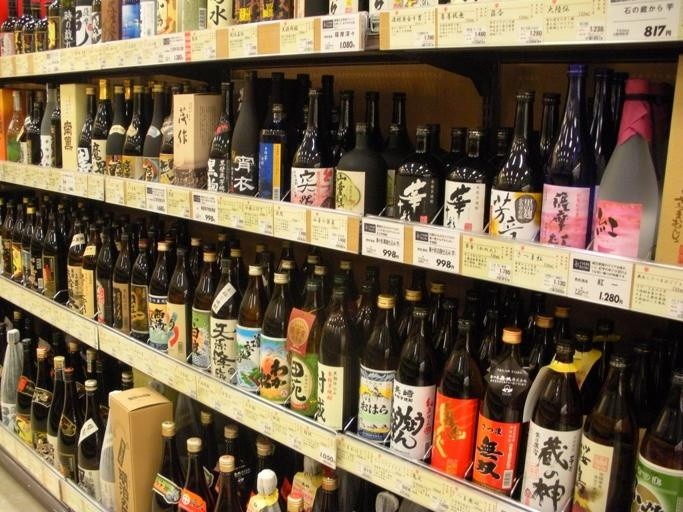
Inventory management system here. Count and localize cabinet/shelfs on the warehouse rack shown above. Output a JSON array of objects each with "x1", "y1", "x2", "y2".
[{"x1": 0, "y1": 0, "x2": 683, "y2": 512}]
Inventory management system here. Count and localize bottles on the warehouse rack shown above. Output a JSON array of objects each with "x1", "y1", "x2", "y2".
[
  {"x1": 0, "y1": 65, "x2": 682, "y2": 512},
  {"x1": 0, "y1": 0, "x2": 101, "y2": 53}
]
